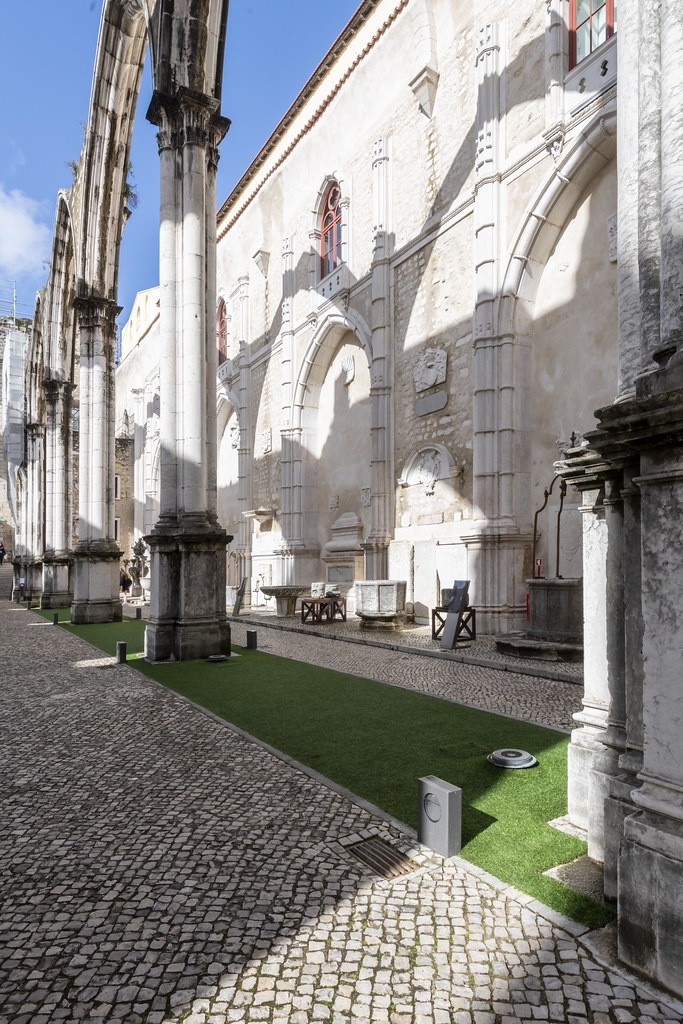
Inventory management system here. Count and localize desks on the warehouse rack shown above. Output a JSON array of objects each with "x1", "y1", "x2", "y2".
[
  {"x1": 302, "y1": 598, "x2": 346, "y2": 624},
  {"x1": 430, "y1": 607, "x2": 477, "y2": 641}
]
